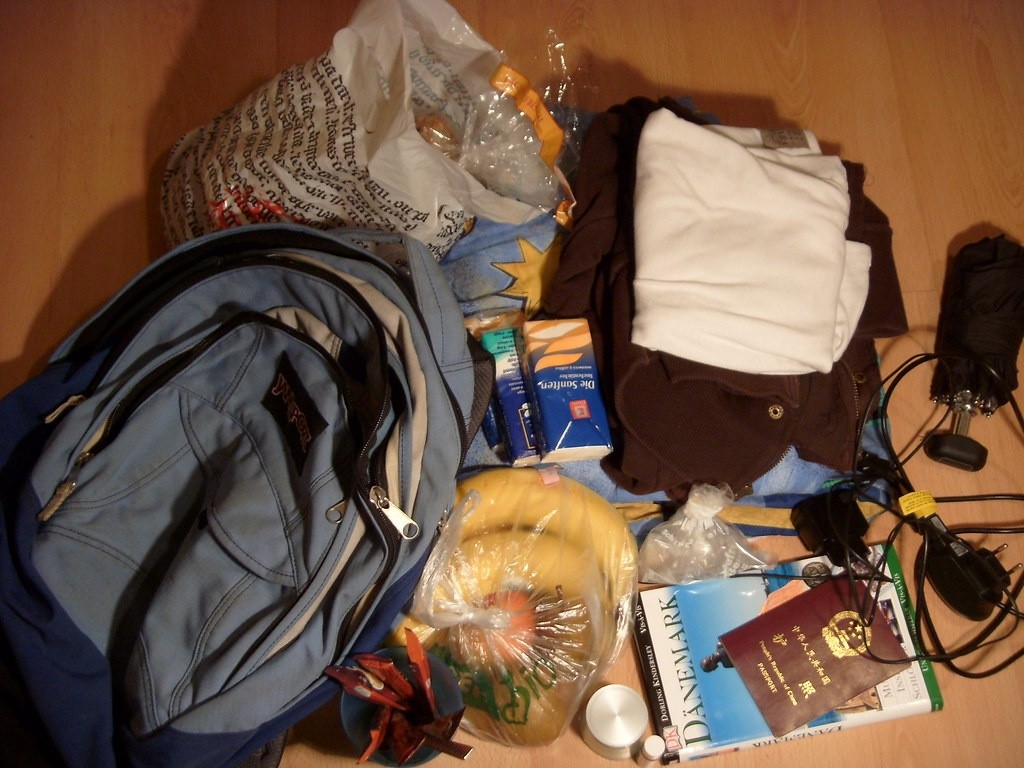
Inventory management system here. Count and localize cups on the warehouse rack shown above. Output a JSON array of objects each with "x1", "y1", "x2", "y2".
[{"x1": 342, "y1": 647, "x2": 462, "y2": 768}]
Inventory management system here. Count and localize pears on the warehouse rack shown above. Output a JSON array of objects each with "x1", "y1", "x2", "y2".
[{"x1": 478, "y1": 583, "x2": 594, "y2": 751}]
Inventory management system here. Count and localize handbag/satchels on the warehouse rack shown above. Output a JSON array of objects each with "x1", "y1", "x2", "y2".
[
  {"x1": 389, "y1": 469, "x2": 641, "y2": 750},
  {"x1": 159, "y1": 0, "x2": 561, "y2": 262}
]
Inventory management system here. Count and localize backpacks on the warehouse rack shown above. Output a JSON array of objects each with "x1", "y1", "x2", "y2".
[{"x1": 0, "y1": 222, "x2": 498, "y2": 767}]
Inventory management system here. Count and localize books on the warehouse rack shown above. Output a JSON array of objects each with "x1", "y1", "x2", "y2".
[{"x1": 634, "y1": 542, "x2": 943, "y2": 763}]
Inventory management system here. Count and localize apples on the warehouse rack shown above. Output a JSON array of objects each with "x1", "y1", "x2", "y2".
[{"x1": 446, "y1": 589, "x2": 537, "y2": 675}]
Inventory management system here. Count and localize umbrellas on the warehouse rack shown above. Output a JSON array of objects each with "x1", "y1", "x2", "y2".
[{"x1": 925, "y1": 237, "x2": 1024, "y2": 472}]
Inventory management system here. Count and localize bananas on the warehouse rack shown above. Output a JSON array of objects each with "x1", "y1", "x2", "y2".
[{"x1": 385, "y1": 466, "x2": 638, "y2": 648}]
